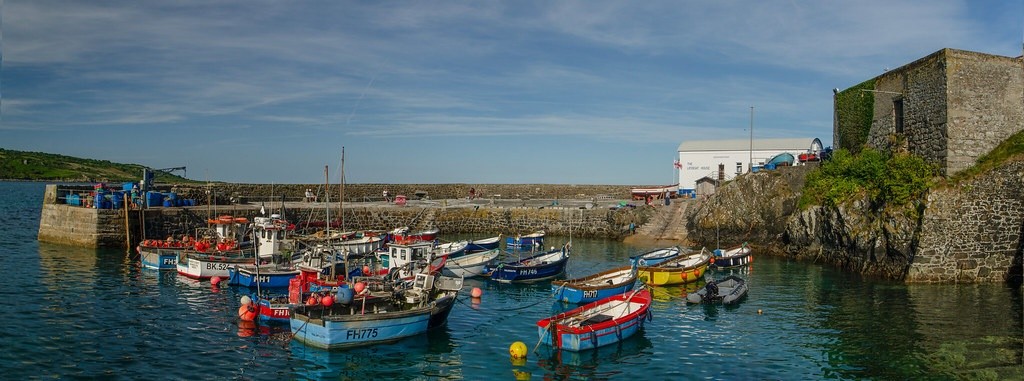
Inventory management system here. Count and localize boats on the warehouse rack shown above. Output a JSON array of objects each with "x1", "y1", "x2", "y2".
[
  {"x1": 636, "y1": 247, "x2": 712, "y2": 286},
  {"x1": 631, "y1": 183, "x2": 680, "y2": 200},
  {"x1": 767, "y1": 152, "x2": 795, "y2": 168},
  {"x1": 712, "y1": 242, "x2": 753, "y2": 269},
  {"x1": 551, "y1": 266, "x2": 639, "y2": 305},
  {"x1": 536, "y1": 288, "x2": 652, "y2": 351},
  {"x1": 486, "y1": 224, "x2": 571, "y2": 286},
  {"x1": 629, "y1": 245, "x2": 680, "y2": 269},
  {"x1": 687, "y1": 275, "x2": 748, "y2": 307},
  {"x1": 506, "y1": 230, "x2": 546, "y2": 250},
  {"x1": 137, "y1": 148, "x2": 503, "y2": 350}
]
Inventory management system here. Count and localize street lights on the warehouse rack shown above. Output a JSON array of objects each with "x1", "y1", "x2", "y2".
[{"x1": 750, "y1": 107, "x2": 755, "y2": 172}]
